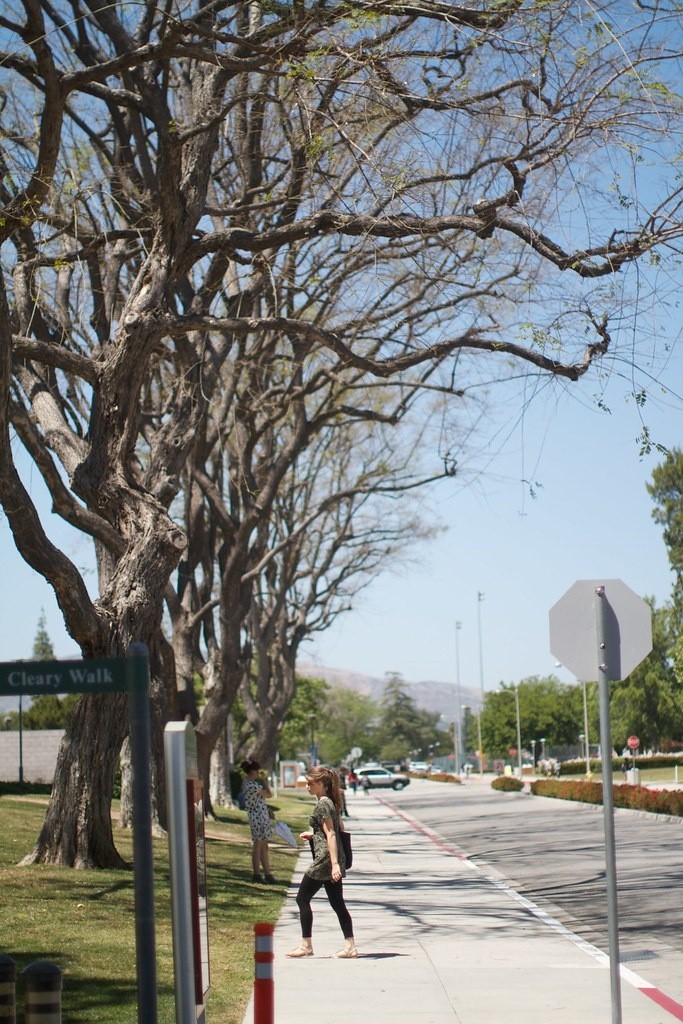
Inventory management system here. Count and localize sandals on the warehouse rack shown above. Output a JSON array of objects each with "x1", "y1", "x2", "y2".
[
  {"x1": 330, "y1": 947, "x2": 358, "y2": 958},
  {"x1": 285, "y1": 945, "x2": 315, "y2": 958}
]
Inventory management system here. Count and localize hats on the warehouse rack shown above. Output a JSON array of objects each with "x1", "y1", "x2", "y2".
[{"x1": 339, "y1": 764, "x2": 349, "y2": 770}]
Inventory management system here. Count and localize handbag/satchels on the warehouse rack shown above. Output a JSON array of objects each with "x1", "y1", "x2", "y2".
[{"x1": 309, "y1": 831, "x2": 352, "y2": 868}]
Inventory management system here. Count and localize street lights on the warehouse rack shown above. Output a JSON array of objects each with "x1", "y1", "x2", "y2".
[
  {"x1": 554, "y1": 659, "x2": 591, "y2": 781},
  {"x1": 470, "y1": 573, "x2": 487, "y2": 777},
  {"x1": 454, "y1": 620, "x2": 465, "y2": 775},
  {"x1": 495, "y1": 687, "x2": 523, "y2": 782}
]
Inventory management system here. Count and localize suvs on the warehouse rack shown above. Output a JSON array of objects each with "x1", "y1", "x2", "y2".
[{"x1": 350, "y1": 766, "x2": 410, "y2": 792}]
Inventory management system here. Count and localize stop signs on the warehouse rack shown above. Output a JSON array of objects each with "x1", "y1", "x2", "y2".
[{"x1": 628, "y1": 735, "x2": 641, "y2": 750}]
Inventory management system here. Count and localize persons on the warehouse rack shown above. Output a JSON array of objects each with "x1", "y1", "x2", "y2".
[
  {"x1": 240, "y1": 760, "x2": 278, "y2": 884},
  {"x1": 622, "y1": 758, "x2": 632, "y2": 773},
  {"x1": 544, "y1": 759, "x2": 561, "y2": 779},
  {"x1": 338, "y1": 767, "x2": 371, "y2": 817},
  {"x1": 285, "y1": 766, "x2": 359, "y2": 959}
]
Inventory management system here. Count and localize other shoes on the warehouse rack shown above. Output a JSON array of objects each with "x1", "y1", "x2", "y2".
[
  {"x1": 251, "y1": 874, "x2": 269, "y2": 885},
  {"x1": 266, "y1": 872, "x2": 279, "y2": 884}
]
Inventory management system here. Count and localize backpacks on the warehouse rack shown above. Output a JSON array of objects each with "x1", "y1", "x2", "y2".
[{"x1": 363, "y1": 777, "x2": 369, "y2": 787}]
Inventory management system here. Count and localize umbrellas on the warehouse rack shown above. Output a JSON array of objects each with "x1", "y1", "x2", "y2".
[{"x1": 274, "y1": 818, "x2": 298, "y2": 850}]
